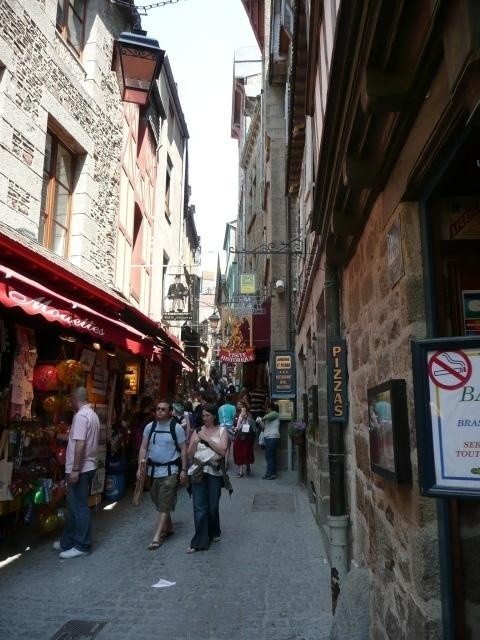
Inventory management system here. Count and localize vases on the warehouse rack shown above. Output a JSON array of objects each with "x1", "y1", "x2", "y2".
[{"x1": 291, "y1": 432, "x2": 306, "y2": 445}]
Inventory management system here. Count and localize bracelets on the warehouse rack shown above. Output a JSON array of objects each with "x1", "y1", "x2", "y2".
[{"x1": 72, "y1": 469, "x2": 81, "y2": 471}]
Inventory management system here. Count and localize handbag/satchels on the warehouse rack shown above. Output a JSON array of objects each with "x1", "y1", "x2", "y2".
[{"x1": 259, "y1": 431, "x2": 265, "y2": 446}]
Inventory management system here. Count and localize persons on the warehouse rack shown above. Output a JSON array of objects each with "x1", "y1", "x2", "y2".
[
  {"x1": 130, "y1": 374, "x2": 281, "y2": 491},
  {"x1": 167, "y1": 275, "x2": 189, "y2": 312},
  {"x1": 135, "y1": 400, "x2": 188, "y2": 550},
  {"x1": 52, "y1": 386, "x2": 101, "y2": 559},
  {"x1": 186, "y1": 405, "x2": 229, "y2": 554}
]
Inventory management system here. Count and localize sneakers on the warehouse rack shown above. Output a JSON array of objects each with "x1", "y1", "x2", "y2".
[{"x1": 52, "y1": 540, "x2": 90, "y2": 559}]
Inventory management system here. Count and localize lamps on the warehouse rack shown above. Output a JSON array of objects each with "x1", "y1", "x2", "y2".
[
  {"x1": 109, "y1": 4, "x2": 167, "y2": 107},
  {"x1": 207, "y1": 307, "x2": 221, "y2": 330}
]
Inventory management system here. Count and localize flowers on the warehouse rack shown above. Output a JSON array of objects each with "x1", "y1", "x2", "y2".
[{"x1": 287, "y1": 418, "x2": 307, "y2": 439}]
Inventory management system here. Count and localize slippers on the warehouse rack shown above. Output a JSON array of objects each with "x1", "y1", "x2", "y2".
[{"x1": 148, "y1": 530, "x2": 175, "y2": 550}]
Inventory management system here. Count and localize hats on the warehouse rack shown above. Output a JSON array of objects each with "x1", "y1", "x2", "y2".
[{"x1": 172, "y1": 403, "x2": 184, "y2": 414}]
